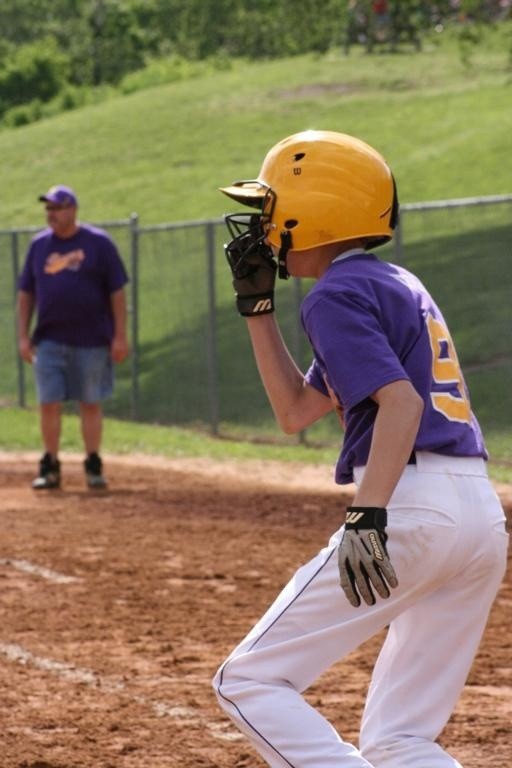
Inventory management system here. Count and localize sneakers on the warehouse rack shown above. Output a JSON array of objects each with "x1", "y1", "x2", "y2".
[
  {"x1": 81, "y1": 453, "x2": 107, "y2": 486},
  {"x1": 33, "y1": 454, "x2": 62, "y2": 488}
]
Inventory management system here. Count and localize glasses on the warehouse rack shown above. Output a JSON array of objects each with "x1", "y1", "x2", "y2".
[{"x1": 43, "y1": 202, "x2": 74, "y2": 210}]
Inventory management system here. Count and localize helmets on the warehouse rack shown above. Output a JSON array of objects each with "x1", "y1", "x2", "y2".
[{"x1": 219, "y1": 129, "x2": 399, "y2": 277}]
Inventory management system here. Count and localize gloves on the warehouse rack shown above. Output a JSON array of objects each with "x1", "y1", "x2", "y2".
[
  {"x1": 337, "y1": 505, "x2": 399, "y2": 606},
  {"x1": 227, "y1": 234, "x2": 276, "y2": 316}
]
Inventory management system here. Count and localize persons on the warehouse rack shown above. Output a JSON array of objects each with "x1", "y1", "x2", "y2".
[
  {"x1": 208, "y1": 129, "x2": 511, "y2": 768},
  {"x1": 16, "y1": 184, "x2": 131, "y2": 491}
]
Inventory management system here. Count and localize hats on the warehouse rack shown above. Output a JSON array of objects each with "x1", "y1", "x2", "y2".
[{"x1": 36, "y1": 184, "x2": 76, "y2": 205}]
